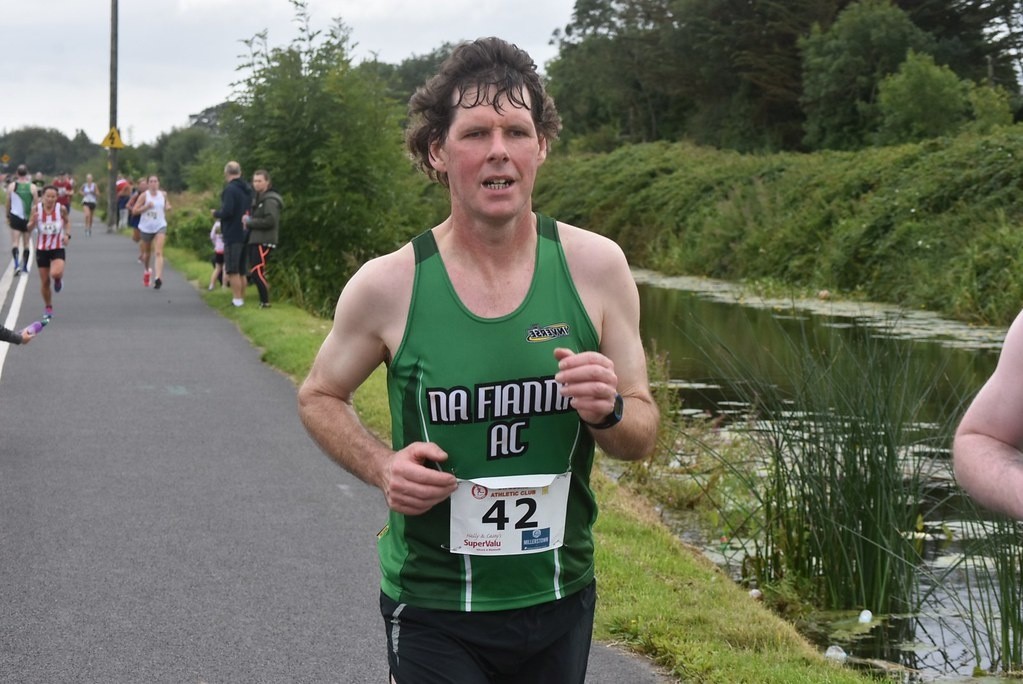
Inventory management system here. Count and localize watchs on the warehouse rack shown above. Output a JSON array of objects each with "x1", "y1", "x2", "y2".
[{"x1": 576, "y1": 392, "x2": 624, "y2": 430}]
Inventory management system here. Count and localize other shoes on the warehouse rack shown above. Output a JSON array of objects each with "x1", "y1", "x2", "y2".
[
  {"x1": 258, "y1": 302, "x2": 269, "y2": 310},
  {"x1": 144, "y1": 267, "x2": 151, "y2": 287},
  {"x1": 154, "y1": 277, "x2": 161, "y2": 289},
  {"x1": 13, "y1": 266, "x2": 21, "y2": 277},
  {"x1": 55, "y1": 278, "x2": 62, "y2": 291},
  {"x1": 43, "y1": 306, "x2": 53, "y2": 319}
]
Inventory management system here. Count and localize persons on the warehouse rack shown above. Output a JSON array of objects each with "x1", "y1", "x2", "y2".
[
  {"x1": 26, "y1": 185, "x2": 71, "y2": 319},
  {"x1": 952, "y1": 305, "x2": 1023, "y2": 522},
  {"x1": 297, "y1": 37, "x2": 659, "y2": 684},
  {"x1": 0, "y1": 164, "x2": 77, "y2": 275},
  {"x1": 209, "y1": 160, "x2": 283, "y2": 309},
  {"x1": 115, "y1": 173, "x2": 172, "y2": 290},
  {"x1": 0, "y1": 324, "x2": 37, "y2": 345},
  {"x1": 79, "y1": 173, "x2": 99, "y2": 237}
]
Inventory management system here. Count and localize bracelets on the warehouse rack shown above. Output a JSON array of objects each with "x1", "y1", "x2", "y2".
[{"x1": 66, "y1": 233, "x2": 71, "y2": 239}]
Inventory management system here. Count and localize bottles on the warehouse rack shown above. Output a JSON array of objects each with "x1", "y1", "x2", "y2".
[{"x1": 15, "y1": 319, "x2": 50, "y2": 339}]
